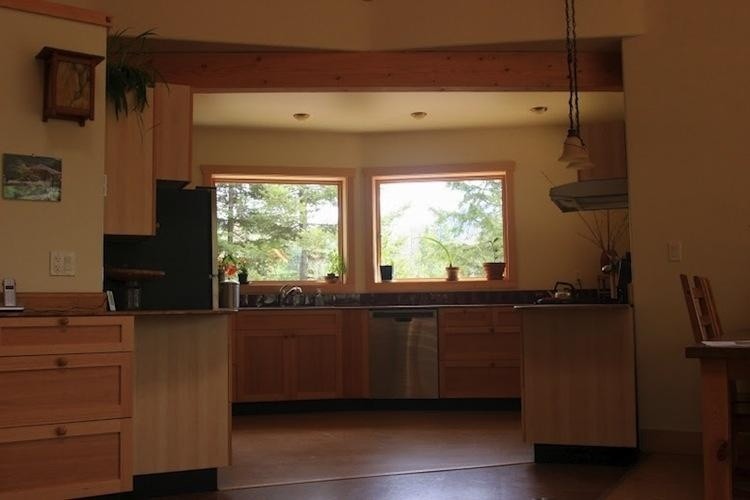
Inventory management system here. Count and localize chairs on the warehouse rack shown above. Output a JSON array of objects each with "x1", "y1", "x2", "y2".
[{"x1": 679, "y1": 272, "x2": 727, "y2": 343}]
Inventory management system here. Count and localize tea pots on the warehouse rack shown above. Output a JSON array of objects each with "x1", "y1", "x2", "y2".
[{"x1": 547, "y1": 281, "x2": 580, "y2": 302}]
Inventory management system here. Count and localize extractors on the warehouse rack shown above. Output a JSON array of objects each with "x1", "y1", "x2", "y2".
[{"x1": 549, "y1": 119, "x2": 629, "y2": 214}]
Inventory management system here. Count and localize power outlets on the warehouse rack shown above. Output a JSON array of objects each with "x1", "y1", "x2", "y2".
[{"x1": 49, "y1": 252, "x2": 64, "y2": 277}]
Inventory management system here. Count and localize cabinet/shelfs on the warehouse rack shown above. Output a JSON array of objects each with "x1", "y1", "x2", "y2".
[
  {"x1": 438, "y1": 305, "x2": 522, "y2": 399},
  {"x1": 234, "y1": 307, "x2": 343, "y2": 404},
  {"x1": 102, "y1": 79, "x2": 161, "y2": 238},
  {"x1": 151, "y1": 80, "x2": 194, "y2": 189},
  {"x1": 135, "y1": 309, "x2": 241, "y2": 475},
  {"x1": 0, "y1": 311, "x2": 137, "y2": 500},
  {"x1": 520, "y1": 306, "x2": 639, "y2": 450}
]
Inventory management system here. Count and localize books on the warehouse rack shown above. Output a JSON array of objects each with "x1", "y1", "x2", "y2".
[{"x1": 0, "y1": 305, "x2": 26, "y2": 312}]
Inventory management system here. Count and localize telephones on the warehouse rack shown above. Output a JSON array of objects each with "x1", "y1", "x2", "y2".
[{"x1": 0, "y1": 279, "x2": 25, "y2": 312}]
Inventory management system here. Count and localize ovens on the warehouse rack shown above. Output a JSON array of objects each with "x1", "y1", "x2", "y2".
[{"x1": 366, "y1": 308, "x2": 439, "y2": 400}]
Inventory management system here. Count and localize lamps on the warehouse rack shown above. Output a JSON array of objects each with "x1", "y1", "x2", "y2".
[
  {"x1": 555, "y1": 0, "x2": 590, "y2": 164},
  {"x1": 564, "y1": 0, "x2": 598, "y2": 171}
]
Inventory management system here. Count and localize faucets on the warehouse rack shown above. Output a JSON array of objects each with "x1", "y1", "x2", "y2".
[{"x1": 277, "y1": 284, "x2": 304, "y2": 306}]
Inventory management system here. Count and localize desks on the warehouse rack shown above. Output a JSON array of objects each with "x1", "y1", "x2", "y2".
[{"x1": 682, "y1": 335, "x2": 749, "y2": 500}]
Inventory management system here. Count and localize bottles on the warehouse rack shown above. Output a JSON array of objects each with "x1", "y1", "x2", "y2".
[
  {"x1": 121, "y1": 279, "x2": 143, "y2": 311},
  {"x1": 220, "y1": 278, "x2": 240, "y2": 309}
]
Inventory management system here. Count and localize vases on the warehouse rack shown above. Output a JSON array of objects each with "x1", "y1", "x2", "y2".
[
  {"x1": 380, "y1": 264, "x2": 393, "y2": 280},
  {"x1": 217, "y1": 269, "x2": 226, "y2": 282}
]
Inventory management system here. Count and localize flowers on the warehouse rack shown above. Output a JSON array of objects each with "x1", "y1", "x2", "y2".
[{"x1": 218, "y1": 251, "x2": 239, "y2": 277}]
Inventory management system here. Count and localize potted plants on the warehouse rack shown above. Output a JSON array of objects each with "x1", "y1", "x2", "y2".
[
  {"x1": 235, "y1": 259, "x2": 252, "y2": 284},
  {"x1": 413, "y1": 231, "x2": 462, "y2": 282},
  {"x1": 480, "y1": 235, "x2": 507, "y2": 281},
  {"x1": 323, "y1": 248, "x2": 349, "y2": 284}
]
